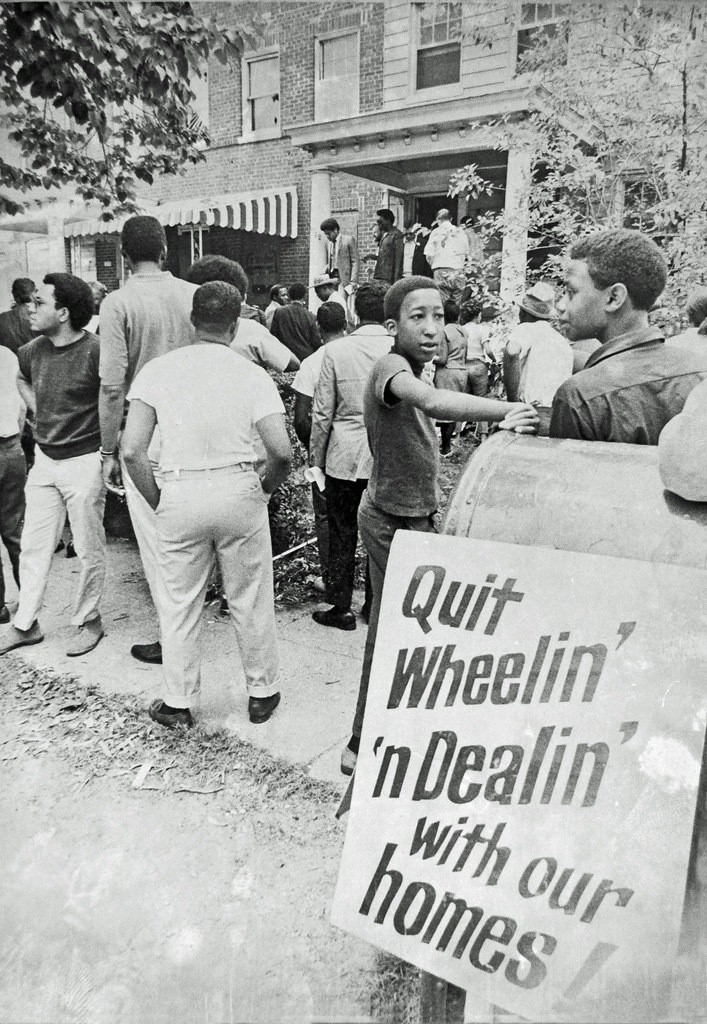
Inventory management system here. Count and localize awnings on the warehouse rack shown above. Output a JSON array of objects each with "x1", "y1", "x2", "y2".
[{"x1": 62, "y1": 187, "x2": 297, "y2": 239}]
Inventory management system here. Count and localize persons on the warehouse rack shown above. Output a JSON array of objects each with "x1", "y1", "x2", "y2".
[
  {"x1": 100, "y1": 216, "x2": 207, "y2": 664},
  {"x1": 191, "y1": 252, "x2": 356, "y2": 374},
  {"x1": 0, "y1": 274, "x2": 107, "y2": 657},
  {"x1": 321, "y1": 219, "x2": 360, "y2": 284},
  {"x1": 369, "y1": 203, "x2": 482, "y2": 279},
  {"x1": 296, "y1": 280, "x2": 395, "y2": 632},
  {"x1": 437, "y1": 223, "x2": 707, "y2": 494},
  {"x1": 120, "y1": 281, "x2": 294, "y2": 730},
  {"x1": 338, "y1": 275, "x2": 541, "y2": 775},
  {"x1": 0, "y1": 344, "x2": 26, "y2": 624}
]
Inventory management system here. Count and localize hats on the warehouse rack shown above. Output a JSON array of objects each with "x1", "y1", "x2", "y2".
[
  {"x1": 307, "y1": 274, "x2": 337, "y2": 289},
  {"x1": 517, "y1": 295, "x2": 557, "y2": 318},
  {"x1": 460, "y1": 215, "x2": 473, "y2": 227},
  {"x1": 526, "y1": 280, "x2": 556, "y2": 301}
]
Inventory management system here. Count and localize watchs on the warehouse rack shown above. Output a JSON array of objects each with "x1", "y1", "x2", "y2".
[{"x1": 98, "y1": 446, "x2": 116, "y2": 457}]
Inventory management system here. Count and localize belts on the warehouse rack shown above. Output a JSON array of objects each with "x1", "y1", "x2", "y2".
[{"x1": 164, "y1": 462, "x2": 255, "y2": 480}]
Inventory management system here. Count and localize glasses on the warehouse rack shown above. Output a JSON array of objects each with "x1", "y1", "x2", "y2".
[
  {"x1": 404, "y1": 231, "x2": 413, "y2": 235},
  {"x1": 29, "y1": 294, "x2": 59, "y2": 308}
]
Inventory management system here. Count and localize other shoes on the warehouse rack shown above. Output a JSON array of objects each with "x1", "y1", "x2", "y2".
[
  {"x1": 66, "y1": 615, "x2": 104, "y2": 656},
  {"x1": 55, "y1": 539, "x2": 64, "y2": 553},
  {"x1": 249, "y1": 692, "x2": 281, "y2": 723},
  {"x1": 149, "y1": 699, "x2": 191, "y2": 726},
  {"x1": 341, "y1": 746, "x2": 357, "y2": 776},
  {"x1": 131, "y1": 641, "x2": 162, "y2": 663},
  {"x1": 438, "y1": 450, "x2": 453, "y2": 457},
  {"x1": 0, "y1": 624, "x2": 44, "y2": 655},
  {"x1": 0, "y1": 607, "x2": 10, "y2": 623},
  {"x1": 67, "y1": 542, "x2": 76, "y2": 557}
]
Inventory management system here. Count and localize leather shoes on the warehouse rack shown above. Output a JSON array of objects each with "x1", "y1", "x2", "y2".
[
  {"x1": 312, "y1": 605, "x2": 357, "y2": 630},
  {"x1": 362, "y1": 602, "x2": 371, "y2": 624}
]
